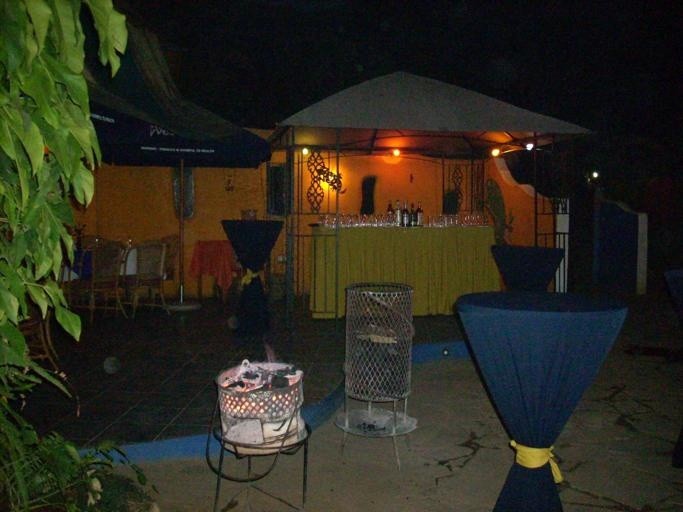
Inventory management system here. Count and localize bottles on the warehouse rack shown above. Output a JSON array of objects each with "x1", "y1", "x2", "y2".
[{"x1": 387, "y1": 199, "x2": 424, "y2": 226}]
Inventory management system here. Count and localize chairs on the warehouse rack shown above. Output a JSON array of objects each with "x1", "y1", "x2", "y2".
[{"x1": 16, "y1": 232, "x2": 180, "y2": 372}]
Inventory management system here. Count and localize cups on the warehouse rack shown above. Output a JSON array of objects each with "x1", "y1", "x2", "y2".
[
  {"x1": 319, "y1": 213, "x2": 483, "y2": 228},
  {"x1": 240, "y1": 209, "x2": 257, "y2": 221}
]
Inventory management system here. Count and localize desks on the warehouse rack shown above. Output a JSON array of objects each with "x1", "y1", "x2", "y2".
[
  {"x1": 311, "y1": 222, "x2": 501, "y2": 317},
  {"x1": 491, "y1": 242, "x2": 566, "y2": 292},
  {"x1": 457, "y1": 290, "x2": 627, "y2": 511},
  {"x1": 189, "y1": 216, "x2": 285, "y2": 337}
]
